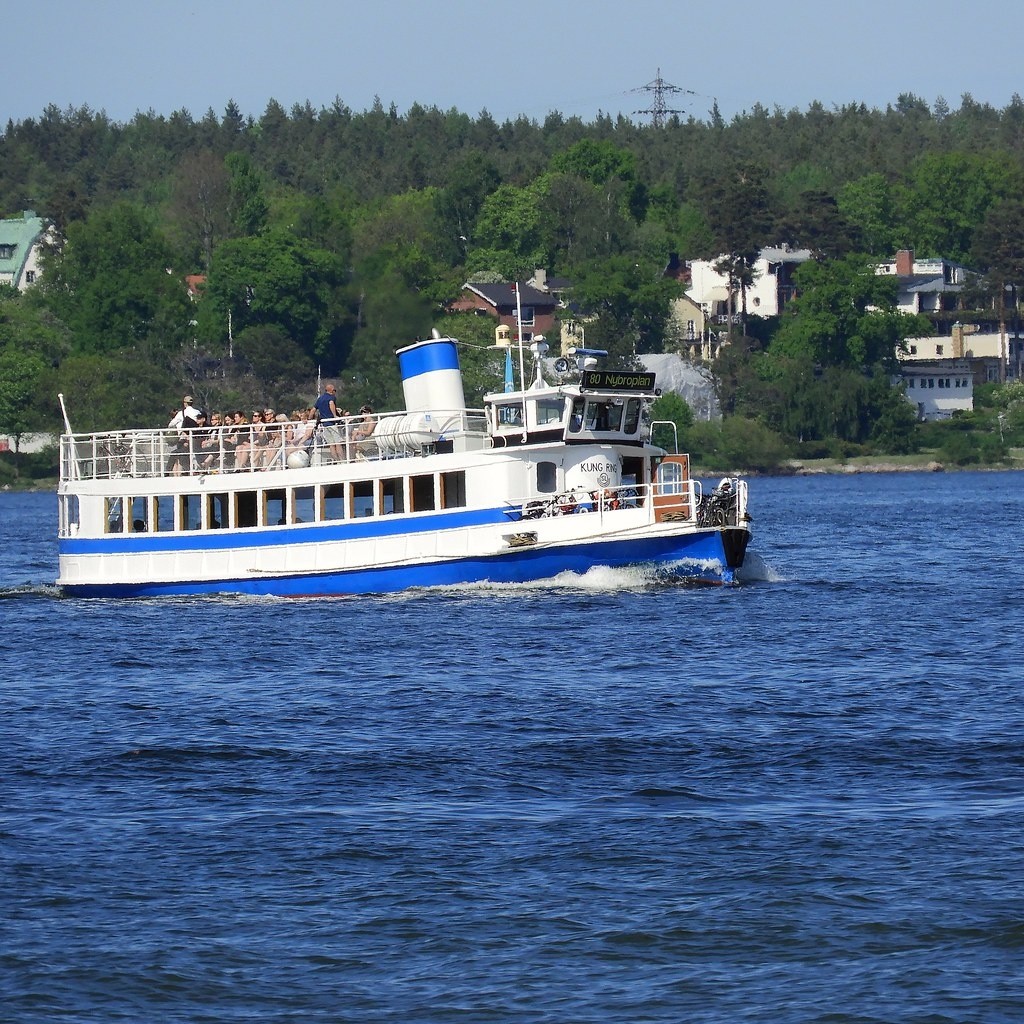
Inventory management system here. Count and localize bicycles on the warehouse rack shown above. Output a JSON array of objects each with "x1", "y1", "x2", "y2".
[
  {"x1": 517, "y1": 486, "x2": 638, "y2": 521},
  {"x1": 694, "y1": 487, "x2": 730, "y2": 527},
  {"x1": 85, "y1": 435, "x2": 148, "y2": 479}
]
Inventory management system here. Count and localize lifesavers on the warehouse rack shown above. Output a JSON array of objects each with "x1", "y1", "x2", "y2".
[{"x1": 593, "y1": 489, "x2": 619, "y2": 512}]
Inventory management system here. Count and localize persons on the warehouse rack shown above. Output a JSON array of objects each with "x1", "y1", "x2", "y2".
[{"x1": 163, "y1": 384, "x2": 377, "y2": 476}]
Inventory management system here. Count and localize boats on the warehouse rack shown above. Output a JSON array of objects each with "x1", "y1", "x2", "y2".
[{"x1": 54, "y1": 278, "x2": 757, "y2": 588}]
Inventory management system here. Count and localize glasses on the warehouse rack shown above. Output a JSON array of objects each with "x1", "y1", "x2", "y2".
[
  {"x1": 361, "y1": 410, "x2": 366, "y2": 413},
  {"x1": 253, "y1": 414, "x2": 260, "y2": 417},
  {"x1": 265, "y1": 413, "x2": 272, "y2": 416},
  {"x1": 211, "y1": 419, "x2": 218, "y2": 421},
  {"x1": 333, "y1": 390, "x2": 336, "y2": 392}
]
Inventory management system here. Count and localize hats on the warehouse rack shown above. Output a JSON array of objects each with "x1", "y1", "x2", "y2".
[
  {"x1": 360, "y1": 406, "x2": 371, "y2": 413},
  {"x1": 183, "y1": 396, "x2": 193, "y2": 403},
  {"x1": 196, "y1": 411, "x2": 207, "y2": 419}
]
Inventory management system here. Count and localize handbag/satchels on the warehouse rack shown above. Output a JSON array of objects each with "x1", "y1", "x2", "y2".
[
  {"x1": 182, "y1": 410, "x2": 200, "y2": 435},
  {"x1": 269, "y1": 437, "x2": 281, "y2": 447},
  {"x1": 258, "y1": 437, "x2": 268, "y2": 446},
  {"x1": 201, "y1": 441, "x2": 212, "y2": 448}
]
